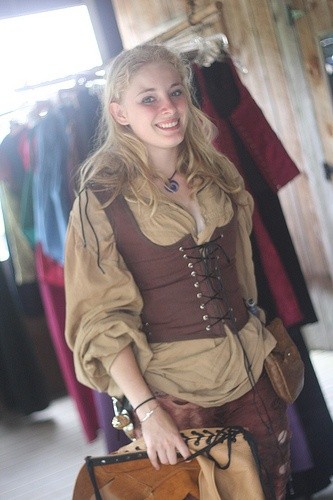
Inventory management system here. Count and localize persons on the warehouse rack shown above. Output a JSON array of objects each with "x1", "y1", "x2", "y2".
[{"x1": 65, "y1": 42, "x2": 294, "y2": 500}]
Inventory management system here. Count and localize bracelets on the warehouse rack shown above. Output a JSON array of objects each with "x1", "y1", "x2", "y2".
[
  {"x1": 131, "y1": 396, "x2": 156, "y2": 411},
  {"x1": 136, "y1": 407, "x2": 160, "y2": 423}
]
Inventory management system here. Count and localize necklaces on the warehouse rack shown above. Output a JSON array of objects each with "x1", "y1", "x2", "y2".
[{"x1": 154, "y1": 170, "x2": 180, "y2": 193}]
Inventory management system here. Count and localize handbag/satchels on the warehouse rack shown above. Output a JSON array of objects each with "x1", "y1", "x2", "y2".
[
  {"x1": 73, "y1": 427, "x2": 269, "y2": 500},
  {"x1": 264, "y1": 317, "x2": 304, "y2": 404}
]
